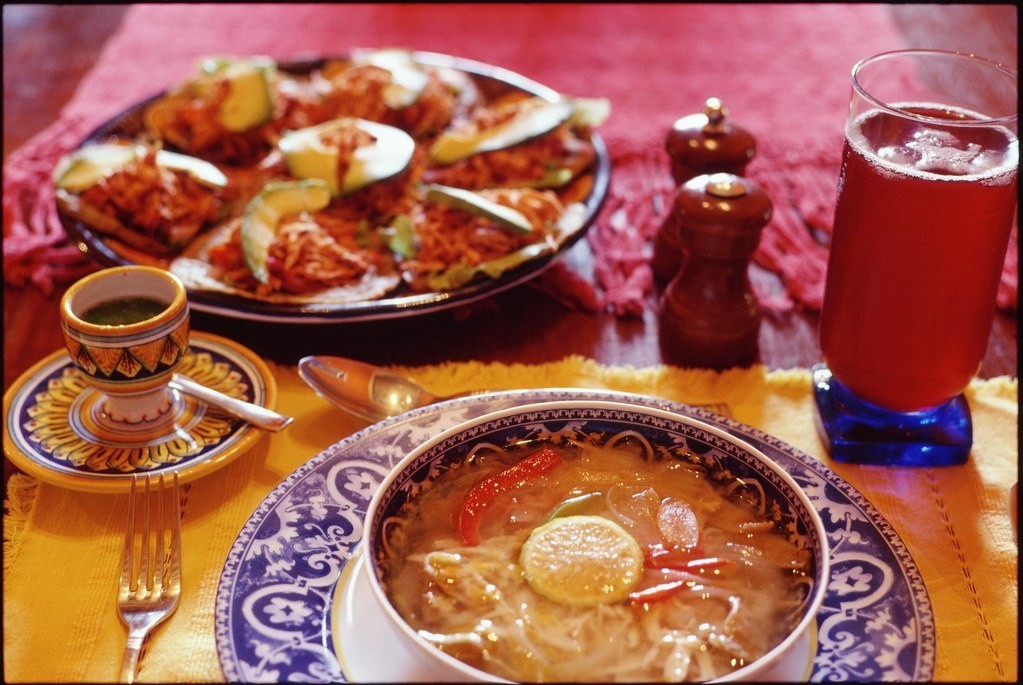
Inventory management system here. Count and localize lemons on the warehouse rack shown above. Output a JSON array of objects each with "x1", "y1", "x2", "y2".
[{"x1": 519, "y1": 513, "x2": 643, "y2": 608}]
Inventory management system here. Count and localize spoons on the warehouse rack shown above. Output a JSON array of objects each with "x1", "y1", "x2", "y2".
[{"x1": 296, "y1": 356, "x2": 731, "y2": 424}]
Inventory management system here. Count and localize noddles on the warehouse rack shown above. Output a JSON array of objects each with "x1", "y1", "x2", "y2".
[{"x1": 389, "y1": 442, "x2": 805, "y2": 685}]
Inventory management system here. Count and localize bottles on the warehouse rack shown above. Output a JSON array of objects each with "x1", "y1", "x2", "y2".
[
  {"x1": 649, "y1": 96, "x2": 756, "y2": 285},
  {"x1": 660, "y1": 172, "x2": 774, "y2": 370}
]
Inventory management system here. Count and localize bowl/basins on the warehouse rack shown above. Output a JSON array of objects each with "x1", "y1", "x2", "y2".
[{"x1": 361, "y1": 398, "x2": 829, "y2": 685}]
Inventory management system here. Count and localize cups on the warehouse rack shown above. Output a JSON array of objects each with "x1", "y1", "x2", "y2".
[
  {"x1": 807, "y1": 48, "x2": 1023, "y2": 413},
  {"x1": 60, "y1": 265, "x2": 191, "y2": 423}
]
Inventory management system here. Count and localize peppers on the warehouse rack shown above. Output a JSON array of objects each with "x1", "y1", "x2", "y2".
[
  {"x1": 457, "y1": 448, "x2": 563, "y2": 546},
  {"x1": 628, "y1": 536, "x2": 735, "y2": 606}
]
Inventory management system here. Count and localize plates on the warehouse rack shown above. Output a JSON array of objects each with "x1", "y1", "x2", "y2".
[
  {"x1": 213, "y1": 388, "x2": 937, "y2": 685},
  {"x1": 52, "y1": 49, "x2": 609, "y2": 331},
  {"x1": 1, "y1": 330, "x2": 277, "y2": 493}
]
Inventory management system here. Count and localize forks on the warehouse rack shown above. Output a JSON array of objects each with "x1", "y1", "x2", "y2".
[{"x1": 116, "y1": 472, "x2": 181, "y2": 684}]
